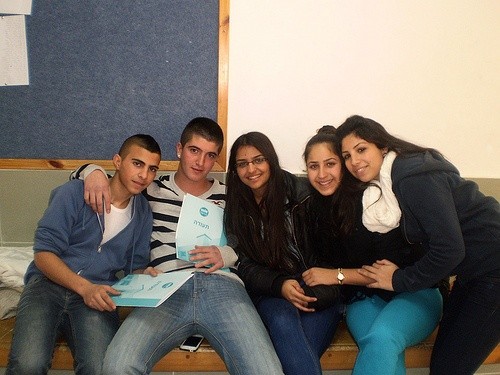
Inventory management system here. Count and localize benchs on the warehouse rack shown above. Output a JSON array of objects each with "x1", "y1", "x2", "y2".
[{"x1": 0, "y1": 317, "x2": 500, "y2": 375}]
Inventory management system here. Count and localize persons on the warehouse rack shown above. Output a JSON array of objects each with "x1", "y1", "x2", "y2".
[
  {"x1": 6, "y1": 134, "x2": 162, "y2": 375},
  {"x1": 302, "y1": 126, "x2": 444, "y2": 375},
  {"x1": 223, "y1": 132, "x2": 340, "y2": 375},
  {"x1": 337, "y1": 115, "x2": 500, "y2": 375},
  {"x1": 69, "y1": 117, "x2": 284, "y2": 374}
]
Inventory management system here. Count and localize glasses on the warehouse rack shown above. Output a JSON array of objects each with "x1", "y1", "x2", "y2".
[{"x1": 236, "y1": 157, "x2": 268, "y2": 168}]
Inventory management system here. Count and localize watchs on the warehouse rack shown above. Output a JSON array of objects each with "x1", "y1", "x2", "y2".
[{"x1": 337, "y1": 268, "x2": 344, "y2": 285}]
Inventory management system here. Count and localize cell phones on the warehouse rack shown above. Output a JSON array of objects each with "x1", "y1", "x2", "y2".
[{"x1": 180, "y1": 333, "x2": 204, "y2": 352}]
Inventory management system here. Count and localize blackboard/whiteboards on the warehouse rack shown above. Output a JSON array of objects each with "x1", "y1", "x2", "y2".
[{"x1": 0, "y1": 0, "x2": 230, "y2": 172}]
